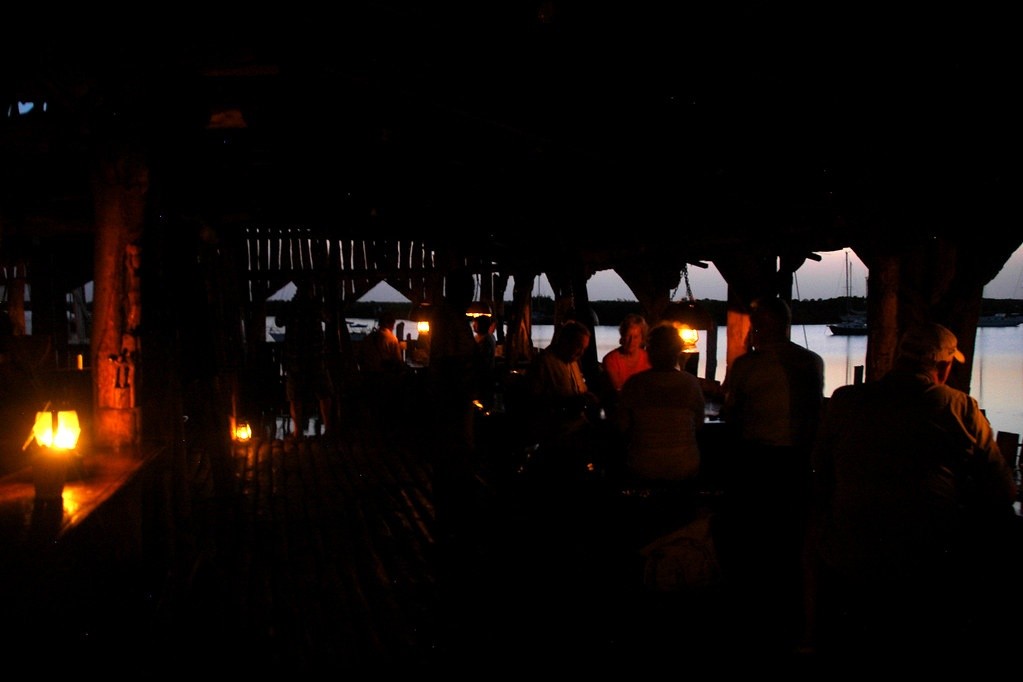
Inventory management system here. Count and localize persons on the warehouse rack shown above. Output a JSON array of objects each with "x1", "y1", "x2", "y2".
[{"x1": 277, "y1": 262, "x2": 1023, "y2": 682}]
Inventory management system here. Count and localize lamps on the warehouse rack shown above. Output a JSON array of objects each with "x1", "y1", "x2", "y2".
[
  {"x1": 465, "y1": 272, "x2": 494, "y2": 318},
  {"x1": 30, "y1": 390, "x2": 83, "y2": 479},
  {"x1": 236, "y1": 417, "x2": 252, "y2": 446}
]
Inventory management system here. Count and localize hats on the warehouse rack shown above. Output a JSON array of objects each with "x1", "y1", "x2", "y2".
[
  {"x1": 899, "y1": 323, "x2": 965, "y2": 363},
  {"x1": 738, "y1": 298, "x2": 792, "y2": 322}
]
